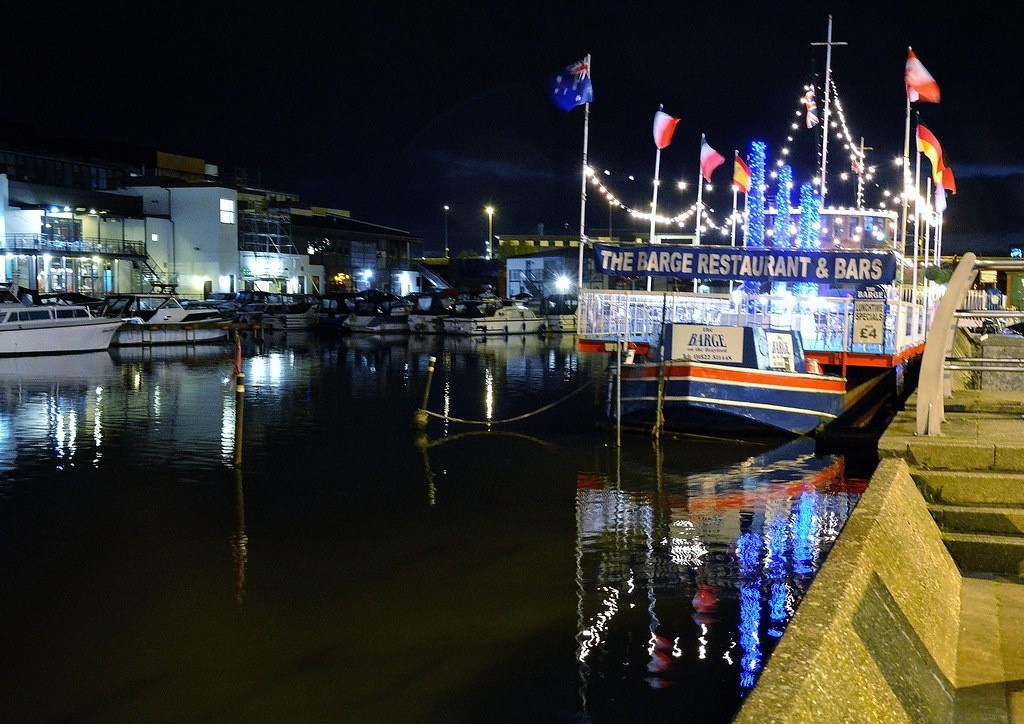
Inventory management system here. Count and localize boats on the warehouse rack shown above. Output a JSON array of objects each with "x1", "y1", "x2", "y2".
[
  {"x1": 603, "y1": 318, "x2": 854, "y2": 436},
  {"x1": 0, "y1": 280, "x2": 580, "y2": 370}
]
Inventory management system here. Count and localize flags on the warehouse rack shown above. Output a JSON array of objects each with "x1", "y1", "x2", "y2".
[
  {"x1": 653, "y1": 111, "x2": 680, "y2": 149},
  {"x1": 806, "y1": 86, "x2": 819, "y2": 128},
  {"x1": 916, "y1": 118, "x2": 944, "y2": 177},
  {"x1": 701, "y1": 138, "x2": 725, "y2": 183},
  {"x1": 551, "y1": 57, "x2": 593, "y2": 111},
  {"x1": 906, "y1": 52, "x2": 940, "y2": 102},
  {"x1": 932, "y1": 165, "x2": 956, "y2": 195},
  {"x1": 733, "y1": 156, "x2": 751, "y2": 193}
]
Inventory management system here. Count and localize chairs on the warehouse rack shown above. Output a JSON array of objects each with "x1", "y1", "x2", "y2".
[
  {"x1": 588, "y1": 298, "x2": 723, "y2": 332},
  {"x1": 813, "y1": 309, "x2": 896, "y2": 354}
]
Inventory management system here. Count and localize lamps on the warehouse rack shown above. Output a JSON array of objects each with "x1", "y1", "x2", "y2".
[{"x1": 111, "y1": 218, "x2": 121, "y2": 222}]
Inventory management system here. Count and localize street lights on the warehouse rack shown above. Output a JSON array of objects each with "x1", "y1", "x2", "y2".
[
  {"x1": 443, "y1": 205, "x2": 451, "y2": 257},
  {"x1": 485, "y1": 204, "x2": 496, "y2": 259}
]
